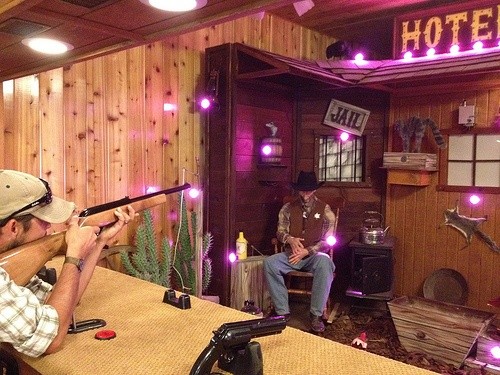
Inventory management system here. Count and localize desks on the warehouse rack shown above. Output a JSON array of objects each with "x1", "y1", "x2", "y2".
[{"x1": 231, "y1": 256, "x2": 273, "y2": 313}]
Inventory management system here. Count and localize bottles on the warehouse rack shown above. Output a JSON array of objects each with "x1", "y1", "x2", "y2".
[{"x1": 236, "y1": 228, "x2": 247, "y2": 259}]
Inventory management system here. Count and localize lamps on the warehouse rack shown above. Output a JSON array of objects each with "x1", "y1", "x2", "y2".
[{"x1": 200, "y1": 67, "x2": 221, "y2": 113}]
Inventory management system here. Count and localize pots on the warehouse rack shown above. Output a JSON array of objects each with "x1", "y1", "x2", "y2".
[{"x1": 423, "y1": 268, "x2": 468, "y2": 306}]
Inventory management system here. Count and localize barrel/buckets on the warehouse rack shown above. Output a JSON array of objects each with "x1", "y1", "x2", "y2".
[{"x1": 260, "y1": 135, "x2": 283, "y2": 165}]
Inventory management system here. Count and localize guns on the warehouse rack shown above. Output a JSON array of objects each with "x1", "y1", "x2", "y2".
[
  {"x1": 0, "y1": 182, "x2": 191, "y2": 287},
  {"x1": 189, "y1": 315, "x2": 286, "y2": 375}
]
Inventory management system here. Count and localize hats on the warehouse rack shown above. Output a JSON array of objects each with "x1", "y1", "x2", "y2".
[
  {"x1": 290, "y1": 170, "x2": 325, "y2": 191},
  {"x1": 0, "y1": 169, "x2": 76, "y2": 223}
]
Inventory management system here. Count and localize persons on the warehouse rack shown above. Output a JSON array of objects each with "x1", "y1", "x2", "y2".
[
  {"x1": 264, "y1": 171, "x2": 336, "y2": 333},
  {"x1": 0, "y1": 170, "x2": 139, "y2": 358}
]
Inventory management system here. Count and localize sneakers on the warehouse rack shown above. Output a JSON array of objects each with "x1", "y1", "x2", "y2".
[
  {"x1": 284, "y1": 313, "x2": 291, "y2": 321},
  {"x1": 309, "y1": 312, "x2": 324, "y2": 331}
]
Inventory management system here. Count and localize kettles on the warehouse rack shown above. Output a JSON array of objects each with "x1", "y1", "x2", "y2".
[{"x1": 360, "y1": 210, "x2": 391, "y2": 245}]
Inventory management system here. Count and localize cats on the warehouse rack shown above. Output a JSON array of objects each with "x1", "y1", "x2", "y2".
[{"x1": 394, "y1": 116, "x2": 447, "y2": 152}]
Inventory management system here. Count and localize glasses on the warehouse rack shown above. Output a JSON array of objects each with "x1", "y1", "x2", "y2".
[{"x1": 0, "y1": 178, "x2": 53, "y2": 227}]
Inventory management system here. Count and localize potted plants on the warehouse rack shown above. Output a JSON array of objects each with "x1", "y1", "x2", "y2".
[{"x1": 120, "y1": 191, "x2": 219, "y2": 305}]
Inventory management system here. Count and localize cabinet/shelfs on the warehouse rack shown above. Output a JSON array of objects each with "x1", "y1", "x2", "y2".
[
  {"x1": 351, "y1": 248, "x2": 392, "y2": 295},
  {"x1": 256, "y1": 164, "x2": 286, "y2": 187}
]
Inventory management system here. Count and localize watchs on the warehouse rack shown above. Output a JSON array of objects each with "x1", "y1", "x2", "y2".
[{"x1": 64, "y1": 257, "x2": 86, "y2": 272}]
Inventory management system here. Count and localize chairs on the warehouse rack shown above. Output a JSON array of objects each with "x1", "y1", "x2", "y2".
[{"x1": 272, "y1": 206, "x2": 340, "y2": 320}]
